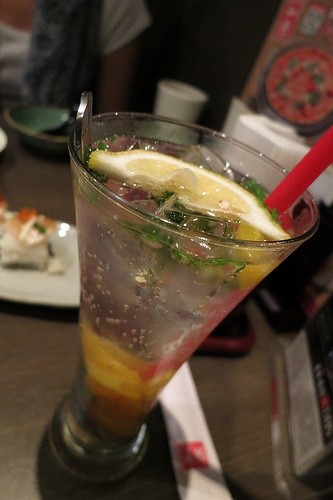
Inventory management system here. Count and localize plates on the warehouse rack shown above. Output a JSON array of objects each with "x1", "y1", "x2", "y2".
[
  {"x1": 1, "y1": 209, "x2": 80, "y2": 308},
  {"x1": 5, "y1": 102, "x2": 78, "y2": 151}
]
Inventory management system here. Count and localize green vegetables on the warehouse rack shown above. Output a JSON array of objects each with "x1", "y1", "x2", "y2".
[{"x1": 85, "y1": 141, "x2": 278, "y2": 285}]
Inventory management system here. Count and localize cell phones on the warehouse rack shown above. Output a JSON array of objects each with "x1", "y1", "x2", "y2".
[{"x1": 194, "y1": 306, "x2": 254, "y2": 356}]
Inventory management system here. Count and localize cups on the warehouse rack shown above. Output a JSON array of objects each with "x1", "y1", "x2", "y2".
[
  {"x1": 48, "y1": 110, "x2": 320, "y2": 485},
  {"x1": 154, "y1": 78, "x2": 209, "y2": 124}
]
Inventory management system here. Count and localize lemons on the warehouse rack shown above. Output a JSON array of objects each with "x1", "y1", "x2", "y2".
[{"x1": 88, "y1": 150, "x2": 291, "y2": 240}]
